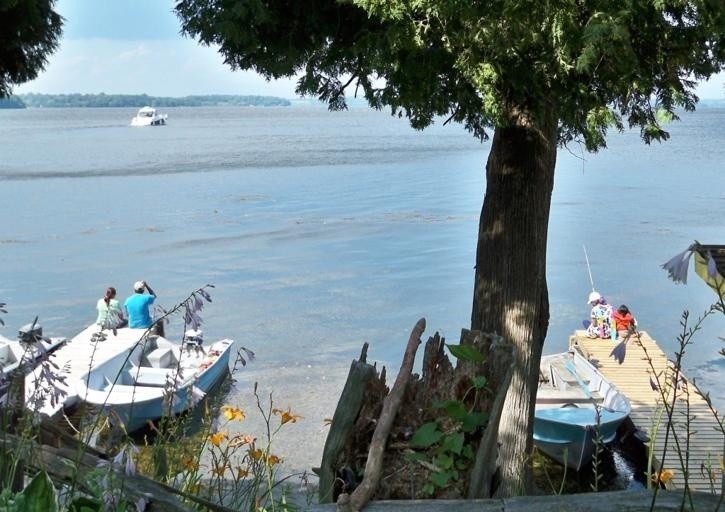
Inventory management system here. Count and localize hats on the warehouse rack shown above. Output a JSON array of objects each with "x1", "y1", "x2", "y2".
[
  {"x1": 134, "y1": 281, "x2": 144, "y2": 290},
  {"x1": 587, "y1": 292, "x2": 600, "y2": 304}
]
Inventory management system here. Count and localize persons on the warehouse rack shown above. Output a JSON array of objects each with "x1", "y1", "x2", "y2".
[
  {"x1": 583, "y1": 291, "x2": 613, "y2": 339},
  {"x1": 96, "y1": 287, "x2": 125, "y2": 329},
  {"x1": 124, "y1": 281, "x2": 158, "y2": 330},
  {"x1": 611, "y1": 304, "x2": 636, "y2": 338}
]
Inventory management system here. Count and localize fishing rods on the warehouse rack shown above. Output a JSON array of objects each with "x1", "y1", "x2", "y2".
[{"x1": 582, "y1": 243, "x2": 594, "y2": 293}]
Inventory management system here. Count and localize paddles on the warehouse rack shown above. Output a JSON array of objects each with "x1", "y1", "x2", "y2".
[{"x1": 566, "y1": 363, "x2": 592, "y2": 399}]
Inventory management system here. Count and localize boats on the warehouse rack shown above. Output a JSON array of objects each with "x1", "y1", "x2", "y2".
[
  {"x1": 0, "y1": 320, "x2": 235, "y2": 449},
  {"x1": 131, "y1": 105, "x2": 168, "y2": 126},
  {"x1": 532, "y1": 350, "x2": 632, "y2": 470}
]
